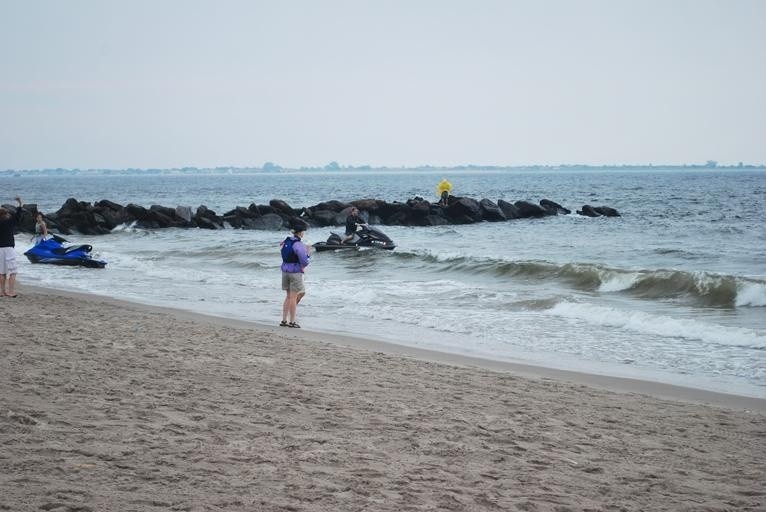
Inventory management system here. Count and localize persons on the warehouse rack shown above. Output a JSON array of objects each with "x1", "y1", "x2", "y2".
[
  {"x1": 278, "y1": 224, "x2": 311, "y2": 328},
  {"x1": 0, "y1": 195, "x2": 22, "y2": 297},
  {"x1": 341, "y1": 207, "x2": 368, "y2": 244},
  {"x1": 439, "y1": 179, "x2": 451, "y2": 207},
  {"x1": 30, "y1": 213, "x2": 48, "y2": 246}
]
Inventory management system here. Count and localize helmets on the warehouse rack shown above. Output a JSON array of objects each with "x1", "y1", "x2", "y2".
[{"x1": 293, "y1": 225, "x2": 307, "y2": 230}]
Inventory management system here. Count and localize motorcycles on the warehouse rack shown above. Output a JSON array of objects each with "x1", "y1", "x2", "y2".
[
  {"x1": 313, "y1": 222, "x2": 396, "y2": 253},
  {"x1": 23, "y1": 235, "x2": 107, "y2": 267}
]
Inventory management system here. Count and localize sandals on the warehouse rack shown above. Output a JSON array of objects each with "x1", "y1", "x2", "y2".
[
  {"x1": 280, "y1": 321, "x2": 288, "y2": 326},
  {"x1": 289, "y1": 322, "x2": 300, "y2": 328}
]
